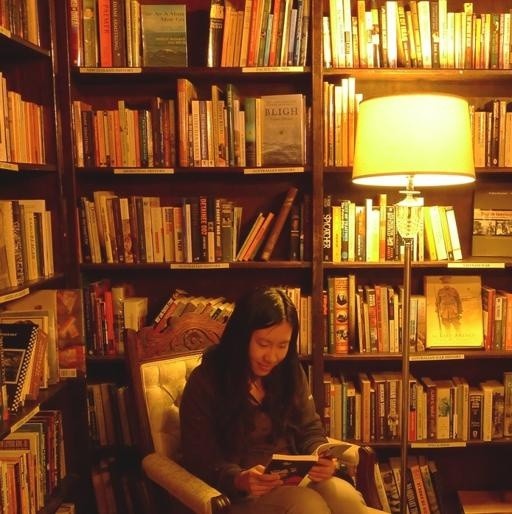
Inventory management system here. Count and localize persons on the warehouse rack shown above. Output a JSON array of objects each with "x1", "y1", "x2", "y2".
[{"x1": 179, "y1": 288, "x2": 370, "y2": 514}]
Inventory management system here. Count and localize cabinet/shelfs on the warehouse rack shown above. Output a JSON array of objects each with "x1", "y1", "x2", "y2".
[{"x1": 0, "y1": 1, "x2": 512, "y2": 514}]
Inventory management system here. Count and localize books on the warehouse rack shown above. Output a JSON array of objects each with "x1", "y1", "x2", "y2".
[
  {"x1": 304, "y1": 362, "x2": 512, "y2": 514},
  {"x1": 322, "y1": 0, "x2": 511, "y2": 354},
  {"x1": 263, "y1": 443, "x2": 352, "y2": 487},
  {"x1": 1, "y1": 357, "x2": 161, "y2": 514},
  {"x1": 1, "y1": 1, "x2": 313, "y2": 357}
]
